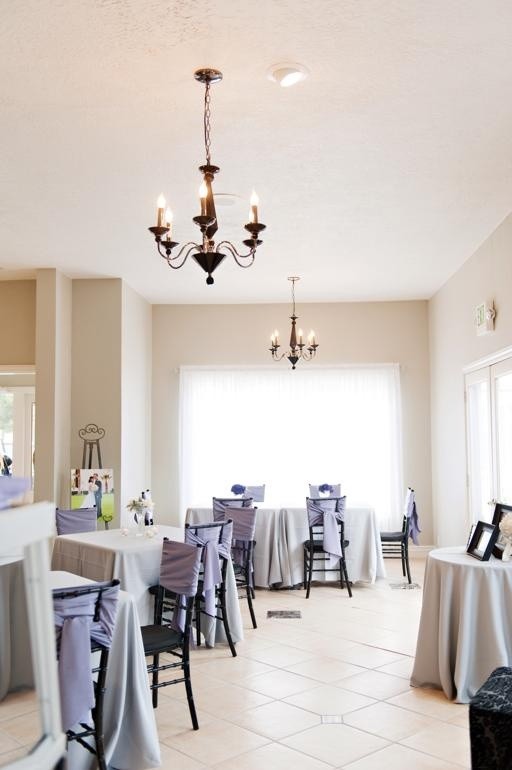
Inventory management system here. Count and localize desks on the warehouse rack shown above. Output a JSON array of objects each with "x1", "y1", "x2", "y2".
[
  {"x1": 185, "y1": 507, "x2": 386, "y2": 589},
  {"x1": 51, "y1": 525, "x2": 185, "y2": 627},
  {"x1": 410, "y1": 541, "x2": 512, "y2": 710},
  {"x1": 50, "y1": 570, "x2": 162, "y2": 770}
]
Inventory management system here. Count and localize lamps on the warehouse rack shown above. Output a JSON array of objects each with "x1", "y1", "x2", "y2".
[
  {"x1": 143, "y1": 69, "x2": 266, "y2": 289},
  {"x1": 266, "y1": 275, "x2": 319, "y2": 371}
]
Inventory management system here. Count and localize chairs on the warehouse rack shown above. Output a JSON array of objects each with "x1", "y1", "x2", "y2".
[
  {"x1": 140, "y1": 537, "x2": 202, "y2": 730},
  {"x1": 149, "y1": 497, "x2": 258, "y2": 657},
  {"x1": 232, "y1": 484, "x2": 265, "y2": 503},
  {"x1": 50, "y1": 578, "x2": 121, "y2": 770},
  {"x1": 55, "y1": 505, "x2": 97, "y2": 535},
  {"x1": 303, "y1": 483, "x2": 353, "y2": 597}
]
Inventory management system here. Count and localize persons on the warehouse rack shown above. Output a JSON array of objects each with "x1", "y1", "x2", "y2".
[{"x1": 80, "y1": 473, "x2": 103, "y2": 519}]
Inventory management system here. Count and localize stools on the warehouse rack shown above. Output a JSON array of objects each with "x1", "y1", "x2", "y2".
[{"x1": 461, "y1": 660, "x2": 512, "y2": 769}]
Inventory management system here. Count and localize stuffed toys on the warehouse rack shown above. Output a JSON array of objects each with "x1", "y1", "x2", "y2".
[{"x1": 499, "y1": 513, "x2": 512, "y2": 562}]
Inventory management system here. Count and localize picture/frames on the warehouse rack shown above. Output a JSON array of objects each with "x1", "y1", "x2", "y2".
[
  {"x1": 490, "y1": 502, "x2": 512, "y2": 560},
  {"x1": 464, "y1": 519, "x2": 500, "y2": 561}
]
